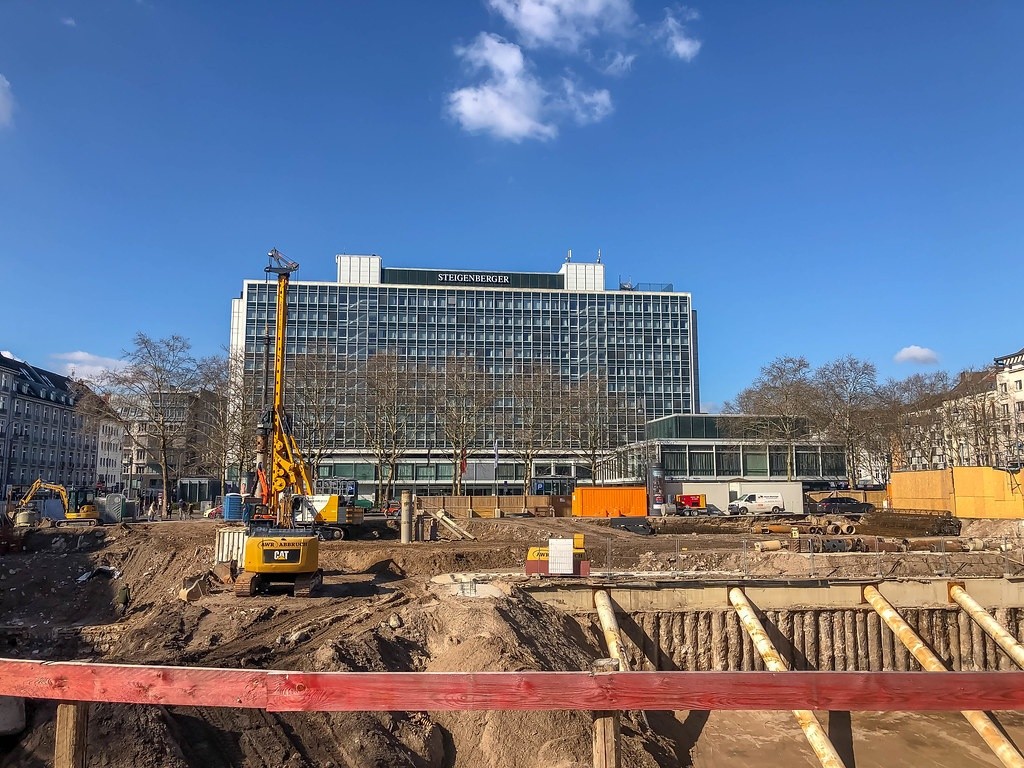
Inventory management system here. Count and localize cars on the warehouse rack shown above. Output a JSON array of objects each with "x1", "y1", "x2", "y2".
[
  {"x1": 380, "y1": 503, "x2": 401, "y2": 517},
  {"x1": 816, "y1": 497, "x2": 877, "y2": 514}
]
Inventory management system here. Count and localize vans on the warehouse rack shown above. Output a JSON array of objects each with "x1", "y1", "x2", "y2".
[{"x1": 728, "y1": 492, "x2": 786, "y2": 515}]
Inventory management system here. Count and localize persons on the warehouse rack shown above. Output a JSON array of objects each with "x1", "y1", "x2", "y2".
[
  {"x1": 147, "y1": 500, "x2": 156, "y2": 522},
  {"x1": 165, "y1": 503, "x2": 172, "y2": 519},
  {"x1": 384, "y1": 499, "x2": 390, "y2": 518},
  {"x1": 118, "y1": 582, "x2": 132, "y2": 616},
  {"x1": 394, "y1": 501, "x2": 402, "y2": 519},
  {"x1": 188, "y1": 502, "x2": 195, "y2": 520},
  {"x1": 178, "y1": 498, "x2": 187, "y2": 521}
]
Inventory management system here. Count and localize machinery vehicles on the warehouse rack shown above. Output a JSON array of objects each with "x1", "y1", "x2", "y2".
[
  {"x1": 3, "y1": 477, "x2": 106, "y2": 527},
  {"x1": 231, "y1": 493, "x2": 324, "y2": 597},
  {"x1": 248, "y1": 247, "x2": 366, "y2": 539}
]
